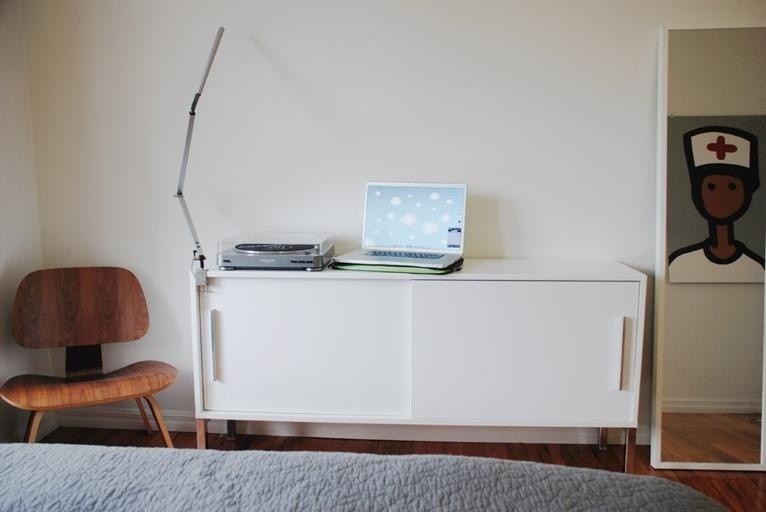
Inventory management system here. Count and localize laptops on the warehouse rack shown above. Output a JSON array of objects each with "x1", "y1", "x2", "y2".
[{"x1": 334, "y1": 181, "x2": 467, "y2": 269}]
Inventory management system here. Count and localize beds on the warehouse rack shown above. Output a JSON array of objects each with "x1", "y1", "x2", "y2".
[{"x1": 1, "y1": 439, "x2": 733, "y2": 512}]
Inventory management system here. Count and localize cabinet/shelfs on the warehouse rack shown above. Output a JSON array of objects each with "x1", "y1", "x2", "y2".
[{"x1": 188, "y1": 259, "x2": 649, "y2": 474}]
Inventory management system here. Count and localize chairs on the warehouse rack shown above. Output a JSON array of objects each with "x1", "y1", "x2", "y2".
[{"x1": 0, "y1": 267, "x2": 178, "y2": 448}]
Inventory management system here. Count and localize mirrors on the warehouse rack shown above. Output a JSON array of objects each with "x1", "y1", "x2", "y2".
[{"x1": 650, "y1": 22, "x2": 765, "y2": 472}]
